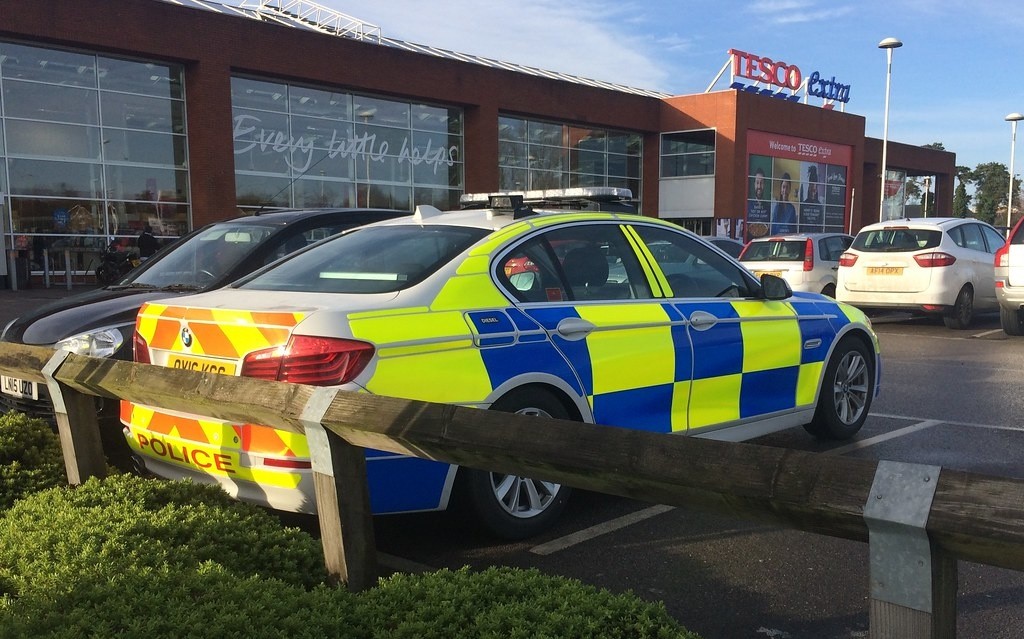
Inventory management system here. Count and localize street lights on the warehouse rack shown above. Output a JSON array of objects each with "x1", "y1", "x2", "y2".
[
  {"x1": 319, "y1": 170, "x2": 325, "y2": 203},
  {"x1": 877, "y1": 37, "x2": 904, "y2": 222},
  {"x1": 1003, "y1": 112, "x2": 1024, "y2": 239},
  {"x1": 923, "y1": 175, "x2": 931, "y2": 218},
  {"x1": 360, "y1": 112, "x2": 374, "y2": 209}
]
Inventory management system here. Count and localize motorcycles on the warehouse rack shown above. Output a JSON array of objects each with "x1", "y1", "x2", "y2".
[{"x1": 95, "y1": 244, "x2": 140, "y2": 288}]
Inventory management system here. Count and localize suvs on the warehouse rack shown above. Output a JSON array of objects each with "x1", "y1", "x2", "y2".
[{"x1": 835, "y1": 217, "x2": 1007, "y2": 330}]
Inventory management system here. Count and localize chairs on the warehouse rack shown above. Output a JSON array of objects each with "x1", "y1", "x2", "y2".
[
  {"x1": 285, "y1": 234, "x2": 308, "y2": 255},
  {"x1": 926, "y1": 231, "x2": 958, "y2": 246},
  {"x1": 893, "y1": 233, "x2": 919, "y2": 248},
  {"x1": 562, "y1": 248, "x2": 609, "y2": 286}
]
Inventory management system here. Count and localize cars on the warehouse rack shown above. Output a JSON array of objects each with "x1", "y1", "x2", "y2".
[
  {"x1": 994, "y1": 214, "x2": 1024, "y2": 334},
  {"x1": 737, "y1": 231, "x2": 855, "y2": 299},
  {"x1": 117, "y1": 182, "x2": 880, "y2": 545},
  {"x1": 0, "y1": 207, "x2": 419, "y2": 435},
  {"x1": 644, "y1": 235, "x2": 746, "y2": 276}
]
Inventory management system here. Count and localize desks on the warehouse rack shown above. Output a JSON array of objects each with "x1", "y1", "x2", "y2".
[{"x1": 49, "y1": 246, "x2": 141, "y2": 258}]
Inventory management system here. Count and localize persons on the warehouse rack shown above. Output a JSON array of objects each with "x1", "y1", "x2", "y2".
[
  {"x1": 138, "y1": 226, "x2": 162, "y2": 262},
  {"x1": 747, "y1": 166, "x2": 824, "y2": 241}
]
